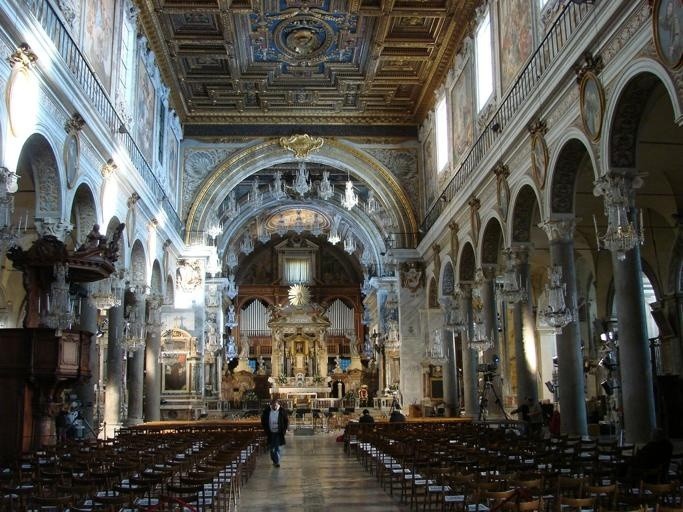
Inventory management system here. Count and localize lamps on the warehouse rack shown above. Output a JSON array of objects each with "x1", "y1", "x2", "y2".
[
  {"x1": 0, "y1": 167, "x2": 177, "y2": 375},
  {"x1": 205, "y1": 162, "x2": 399, "y2": 362},
  {"x1": 594, "y1": 170, "x2": 645, "y2": 262},
  {"x1": 427, "y1": 326, "x2": 449, "y2": 366},
  {"x1": 538, "y1": 262, "x2": 575, "y2": 329},
  {"x1": 494, "y1": 245, "x2": 526, "y2": 304},
  {"x1": 466, "y1": 320, "x2": 491, "y2": 352}
]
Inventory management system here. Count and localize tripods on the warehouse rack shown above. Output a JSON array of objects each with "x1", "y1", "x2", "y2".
[
  {"x1": 478, "y1": 375, "x2": 508, "y2": 421},
  {"x1": 390, "y1": 400, "x2": 400, "y2": 411}
]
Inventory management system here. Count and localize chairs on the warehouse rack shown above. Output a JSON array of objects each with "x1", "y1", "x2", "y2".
[
  {"x1": 344, "y1": 421, "x2": 683, "y2": 512},
  {"x1": 0, "y1": 426, "x2": 269, "y2": 512}
]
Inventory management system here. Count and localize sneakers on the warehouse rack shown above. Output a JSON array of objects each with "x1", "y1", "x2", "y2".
[{"x1": 275, "y1": 463, "x2": 280, "y2": 467}]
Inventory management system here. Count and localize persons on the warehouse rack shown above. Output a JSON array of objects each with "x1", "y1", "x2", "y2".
[
  {"x1": 261, "y1": 399, "x2": 288, "y2": 468},
  {"x1": 510, "y1": 397, "x2": 561, "y2": 433},
  {"x1": 87, "y1": 224, "x2": 104, "y2": 250},
  {"x1": 359, "y1": 409, "x2": 374, "y2": 423},
  {"x1": 390, "y1": 404, "x2": 406, "y2": 422},
  {"x1": 621, "y1": 428, "x2": 675, "y2": 485}
]
